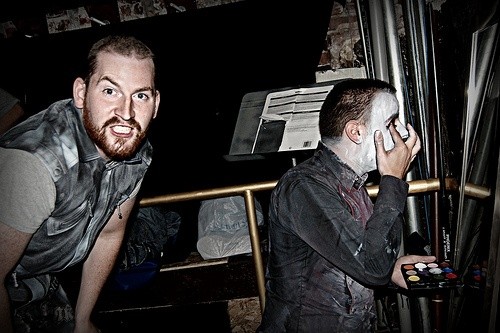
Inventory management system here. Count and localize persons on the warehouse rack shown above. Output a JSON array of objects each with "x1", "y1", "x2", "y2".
[
  {"x1": 254, "y1": 76, "x2": 445, "y2": 333},
  {"x1": 0, "y1": 32, "x2": 164, "y2": 333},
  {"x1": 0, "y1": 89, "x2": 25, "y2": 134}
]
